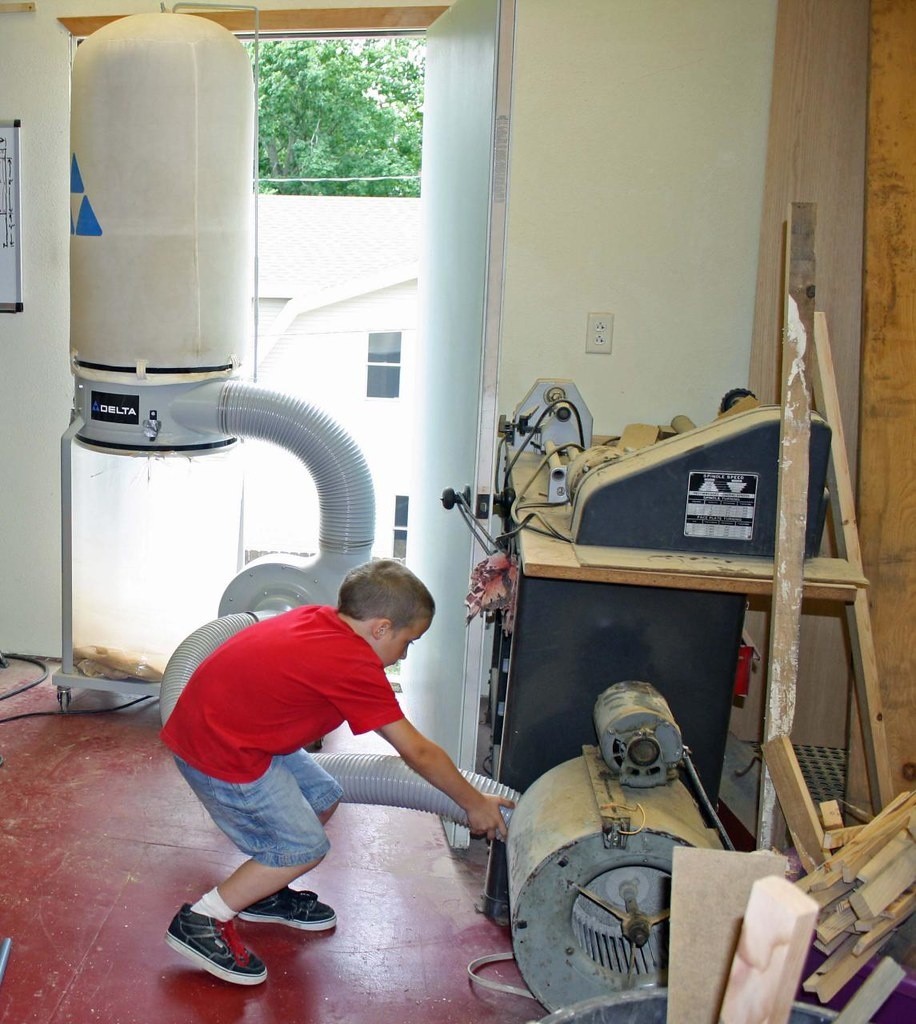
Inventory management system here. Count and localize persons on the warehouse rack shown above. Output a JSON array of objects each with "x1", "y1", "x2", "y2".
[{"x1": 159, "y1": 560, "x2": 518, "y2": 985}]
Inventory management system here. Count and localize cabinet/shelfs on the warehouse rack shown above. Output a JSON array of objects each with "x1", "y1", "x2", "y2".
[{"x1": 481, "y1": 511, "x2": 748, "y2": 918}]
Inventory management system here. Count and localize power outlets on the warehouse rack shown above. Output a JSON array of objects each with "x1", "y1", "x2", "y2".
[{"x1": 585, "y1": 312, "x2": 614, "y2": 355}]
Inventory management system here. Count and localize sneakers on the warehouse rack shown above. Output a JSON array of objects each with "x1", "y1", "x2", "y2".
[
  {"x1": 163, "y1": 902, "x2": 267, "y2": 985},
  {"x1": 237, "y1": 886, "x2": 337, "y2": 931}
]
